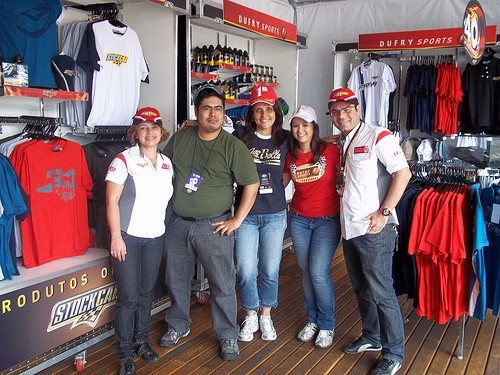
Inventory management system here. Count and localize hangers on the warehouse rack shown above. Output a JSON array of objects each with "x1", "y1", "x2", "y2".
[
  {"x1": 0, "y1": 116, "x2": 78, "y2": 150},
  {"x1": 64, "y1": 3, "x2": 135, "y2": 33},
  {"x1": 405, "y1": 160, "x2": 500, "y2": 201},
  {"x1": 387, "y1": 121, "x2": 442, "y2": 143},
  {"x1": 354, "y1": 52, "x2": 388, "y2": 69},
  {"x1": 468, "y1": 46, "x2": 500, "y2": 65},
  {"x1": 78, "y1": 125, "x2": 132, "y2": 155},
  {"x1": 409, "y1": 55, "x2": 456, "y2": 68}
]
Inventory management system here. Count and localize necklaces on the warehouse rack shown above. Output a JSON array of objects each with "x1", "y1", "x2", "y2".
[{"x1": 299, "y1": 149, "x2": 313, "y2": 161}]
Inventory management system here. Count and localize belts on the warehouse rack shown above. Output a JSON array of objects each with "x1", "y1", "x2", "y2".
[{"x1": 173, "y1": 209, "x2": 231, "y2": 222}]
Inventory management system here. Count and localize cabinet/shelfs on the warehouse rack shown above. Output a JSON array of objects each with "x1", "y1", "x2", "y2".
[{"x1": 192, "y1": 62, "x2": 279, "y2": 111}]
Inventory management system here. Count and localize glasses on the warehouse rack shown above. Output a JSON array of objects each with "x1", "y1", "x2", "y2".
[
  {"x1": 198, "y1": 106, "x2": 224, "y2": 114},
  {"x1": 330, "y1": 105, "x2": 355, "y2": 117}
]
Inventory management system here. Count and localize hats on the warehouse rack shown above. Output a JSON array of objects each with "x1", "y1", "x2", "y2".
[
  {"x1": 328, "y1": 88, "x2": 359, "y2": 105},
  {"x1": 288, "y1": 105, "x2": 318, "y2": 124},
  {"x1": 52, "y1": 55, "x2": 75, "y2": 92},
  {"x1": 132, "y1": 107, "x2": 162, "y2": 125},
  {"x1": 249, "y1": 83, "x2": 278, "y2": 106},
  {"x1": 193, "y1": 84, "x2": 221, "y2": 102}
]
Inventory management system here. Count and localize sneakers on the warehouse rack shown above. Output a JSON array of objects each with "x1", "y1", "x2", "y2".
[
  {"x1": 344, "y1": 337, "x2": 382, "y2": 354},
  {"x1": 314, "y1": 329, "x2": 335, "y2": 349},
  {"x1": 220, "y1": 338, "x2": 239, "y2": 361},
  {"x1": 371, "y1": 358, "x2": 401, "y2": 375},
  {"x1": 238, "y1": 314, "x2": 259, "y2": 341},
  {"x1": 259, "y1": 314, "x2": 277, "y2": 341},
  {"x1": 160, "y1": 326, "x2": 190, "y2": 347},
  {"x1": 134, "y1": 342, "x2": 159, "y2": 362},
  {"x1": 298, "y1": 323, "x2": 320, "y2": 343},
  {"x1": 120, "y1": 357, "x2": 136, "y2": 375}
]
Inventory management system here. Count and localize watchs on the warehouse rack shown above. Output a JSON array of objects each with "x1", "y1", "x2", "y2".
[{"x1": 380, "y1": 208, "x2": 393, "y2": 217}]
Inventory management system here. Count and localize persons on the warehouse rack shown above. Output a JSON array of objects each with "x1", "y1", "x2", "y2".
[
  {"x1": 178, "y1": 83, "x2": 338, "y2": 342},
  {"x1": 159, "y1": 84, "x2": 261, "y2": 360},
  {"x1": 105, "y1": 107, "x2": 174, "y2": 375},
  {"x1": 328, "y1": 88, "x2": 412, "y2": 375},
  {"x1": 282, "y1": 106, "x2": 341, "y2": 348}
]
